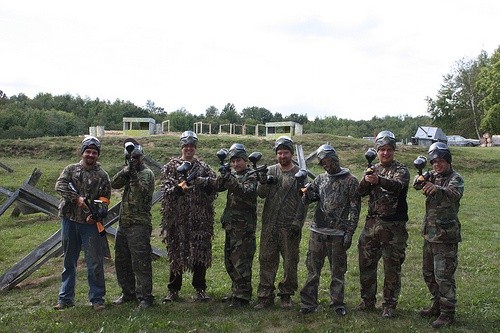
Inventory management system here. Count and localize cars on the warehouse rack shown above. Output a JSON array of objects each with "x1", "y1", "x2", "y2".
[
  {"x1": 491, "y1": 134, "x2": 500, "y2": 146},
  {"x1": 446, "y1": 134, "x2": 481, "y2": 147}
]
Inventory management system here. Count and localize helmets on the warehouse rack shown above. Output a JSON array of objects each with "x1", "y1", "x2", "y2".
[
  {"x1": 429, "y1": 141, "x2": 452, "y2": 164},
  {"x1": 179, "y1": 130, "x2": 198, "y2": 149},
  {"x1": 81, "y1": 136, "x2": 101, "y2": 154},
  {"x1": 229, "y1": 143, "x2": 248, "y2": 161},
  {"x1": 318, "y1": 144, "x2": 336, "y2": 164},
  {"x1": 276, "y1": 136, "x2": 294, "y2": 156},
  {"x1": 376, "y1": 130, "x2": 396, "y2": 151}
]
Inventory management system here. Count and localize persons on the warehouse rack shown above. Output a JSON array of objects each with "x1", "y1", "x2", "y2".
[
  {"x1": 110, "y1": 139, "x2": 156, "y2": 308},
  {"x1": 55, "y1": 136, "x2": 111, "y2": 311},
  {"x1": 298, "y1": 145, "x2": 361, "y2": 316},
  {"x1": 215, "y1": 143, "x2": 258, "y2": 309},
  {"x1": 255, "y1": 135, "x2": 309, "y2": 311},
  {"x1": 352, "y1": 130, "x2": 411, "y2": 318},
  {"x1": 413, "y1": 142, "x2": 465, "y2": 330},
  {"x1": 160, "y1": 131, "x2": 219, "y2": 302}
]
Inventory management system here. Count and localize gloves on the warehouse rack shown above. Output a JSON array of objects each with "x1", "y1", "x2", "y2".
[
  {"x1": 258, "y1": 169, "x2": 268, "y2": 185},
  {"x1": 289, "y1": 226, "x2": 301, "y2": 239},
  {"x1": 123, "y1": 164, "x2": 138, "y2": 178},
  {"x1": 343, "y1": 233, "x2": 352, "y2": 251}
]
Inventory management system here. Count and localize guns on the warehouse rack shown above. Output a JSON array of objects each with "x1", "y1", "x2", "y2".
[
  {"x1": 295, "y1": 168, "x2": 314, "y2": 201},
  {"x1": 412, "y1": 155, "x2": 431, "y2": 192},
  {"x1": 125, "y1": 139, "x2": 134, "y2": 165},
  {"x1": 247, "y1": 150, "x2": 269, "y2": 186},
  {"x1": 68, "y1": 182, "x2": 107, "y2": 236},
  {"x1": 364, "y1": 148, "x2": 378, "y2": 189},
  {"x1": 216, "y1": 146, "x2": 231, "y2": 184},
  {"x1": 172, "y1": 160, "x2": 199, "y2": 192}
]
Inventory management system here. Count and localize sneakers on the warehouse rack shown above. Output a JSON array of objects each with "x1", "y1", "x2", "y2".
[
  {"x1": 193, "y1": 287, "x2": 209, "y2": 300},
  {"x1": 432, "y1": 313, "x2": 454, "y2": 328},
  {"x1": 351, "y1": 298, "x2": 375, "y2": 312},
  {"x1": 162, "y1": 290, "x2": 178, "y2": 302},
  {"x1": 381, "y1": 306, "x2": 394, "y2": 317},
  {"x1": 420, "y1": 302, "x2": 440, "y2": 318},
  {"x1": 253, "y1": 298, "x2": 274, "y2": 311},
  {"x1": 280, "y1": 296, "x2": 293, "y2": 309}
]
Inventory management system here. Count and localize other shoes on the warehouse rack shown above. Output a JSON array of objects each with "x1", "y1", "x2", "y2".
[
  {"x1": 222, "y1": 294, "x2": 250, "y2": 308},
  {"x1": 137, "y1": 294, "x2": 155, "y2": 308},
  {"x1": 335, "y1": 307, "x2": 346, "y2": 317},
  {"x1": 54, "y1": 303, "x2": 74, "y2": 309},
  {"x1": 299, "y1": 308, "x2": 311, "y2": 314},
  {"x1": 112, "y1": 290, "x2": 137, "y2": 304},
  {"x1": 93, "y1": 302, "x2": 105, "y2": 311}
]
